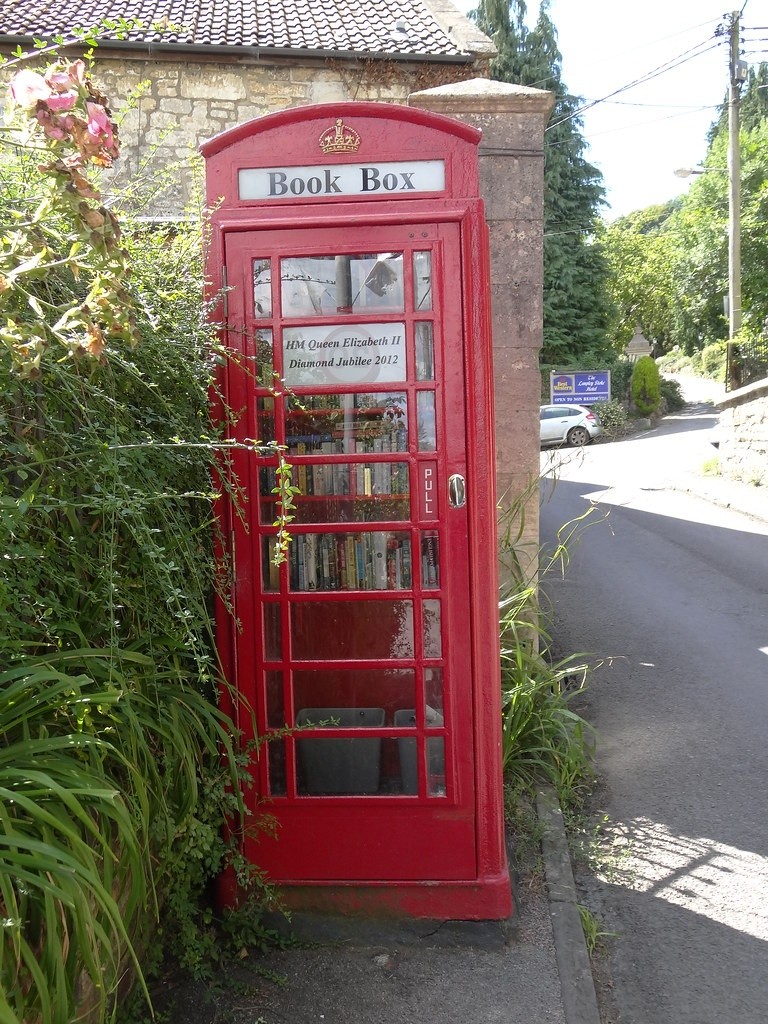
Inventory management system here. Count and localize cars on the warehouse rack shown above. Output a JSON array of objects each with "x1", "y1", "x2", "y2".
[{"x1": 539, "y1": 404, "x2": 604, "y2": 448}]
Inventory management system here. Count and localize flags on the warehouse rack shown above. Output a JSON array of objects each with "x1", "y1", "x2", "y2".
[{"x1": 366, "y1": 261, "x2": 398, "y2": 296}]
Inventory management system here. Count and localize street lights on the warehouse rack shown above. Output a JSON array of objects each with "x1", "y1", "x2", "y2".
[{"x1": 672, "y1": 165, "x2": 744, "y2": 390}]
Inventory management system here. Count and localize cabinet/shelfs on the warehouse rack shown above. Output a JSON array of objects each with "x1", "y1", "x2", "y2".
[{"x1": 256, "y1": 308, "x2": 447, "y2": 778}]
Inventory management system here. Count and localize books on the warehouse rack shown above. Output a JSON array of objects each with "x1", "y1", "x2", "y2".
[{"x1": 261, "y1": 363, "x2": 442, "y2": 589}]
